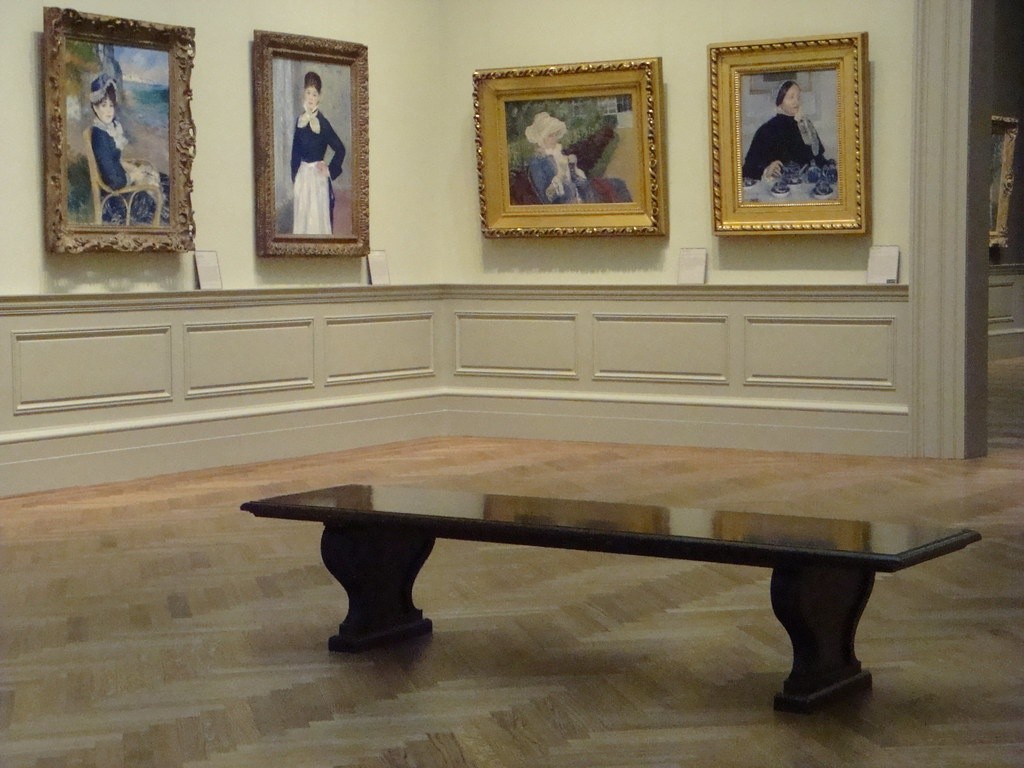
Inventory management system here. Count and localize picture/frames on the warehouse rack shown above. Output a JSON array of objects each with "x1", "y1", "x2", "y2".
[
  {"x1": 988, "y1": 115, "x2": 1019, "y2": 252},
  {"x1": 473, "y1": 57, "x2": 670, "y2": 239},
  {"x1": 707, "y1": 32, "x2": 873, "y2": 237},
  {"x1": 42, "y1": 6, "x2": 197, "y2": 253},
  {"x1": 251, "y1": 29, "x2": 371, "y2": 257}
]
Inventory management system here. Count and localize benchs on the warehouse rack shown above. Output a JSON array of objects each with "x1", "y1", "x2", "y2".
[{"x1": 240, "y1": 484, "x2": 983, "y2": 714}]
